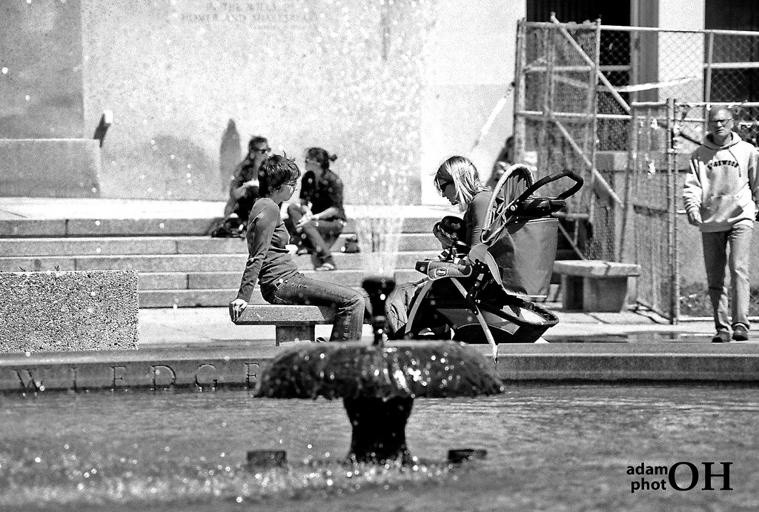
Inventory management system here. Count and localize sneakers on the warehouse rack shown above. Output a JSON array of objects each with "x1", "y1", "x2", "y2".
[
  {"x1": 297, "y1": 248, "x2": 335, "y2": 271},
  {"x1": 713, "y1": 329, "x2": 731, "y2": 342},
  {"x1": 732, "y1": 326, "x2": 749, "y2": 341}
]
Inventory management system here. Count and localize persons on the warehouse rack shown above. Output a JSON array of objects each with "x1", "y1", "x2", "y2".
[
  {"x1": 682, "y1": 106, "x2": 758, "y2": 343},
  {"x1": 223, "y1": 136, "x2": 282, "y2": 227},
  {"x1": 286, "y1": 148, "x2": 345, "y2": 271},
  {"x1": 382, "y1": 156, "x2": 520, "y2": 339},
  {"x1": 230, "y1": 152, "x2": 364, "y2": 342}
]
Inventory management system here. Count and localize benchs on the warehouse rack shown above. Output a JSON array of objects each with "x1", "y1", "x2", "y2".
[
  {"x1": 234, "y1": 303, "x2": 339, "y2": 346},
  {"x1": 554, "y1": 259, "x2": 642, "y2": 314},
  {"x1": 392, "y1": 270, "x2": 549, "y2": 344}
]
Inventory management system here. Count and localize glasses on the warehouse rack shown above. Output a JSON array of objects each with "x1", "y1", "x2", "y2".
[
  {"x1": 257, "y1": 148, "x2": 272, "y2": 153},
  {"x1": 304, "y1": 159, "x2": 318, "y2": 166},
  {"x1": 440, "y1": 181, "x2": 454, "y2": 193}
]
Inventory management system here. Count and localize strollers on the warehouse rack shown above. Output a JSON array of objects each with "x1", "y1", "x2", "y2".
[{"x1": 387, "y1": 164, "x2": 584, "y2": 343}]
